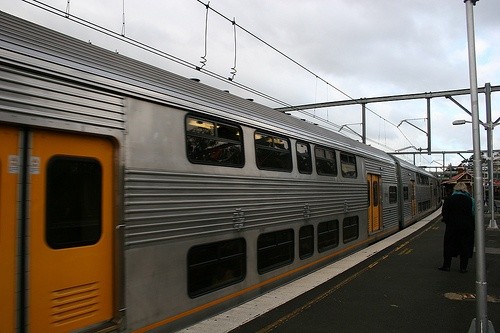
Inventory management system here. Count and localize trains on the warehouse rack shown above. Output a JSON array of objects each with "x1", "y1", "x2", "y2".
[{"x1": 0, "y1": 9, "x2": 443, "y2": 332}]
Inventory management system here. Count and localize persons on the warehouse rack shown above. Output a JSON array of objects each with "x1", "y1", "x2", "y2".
[{"x1": 435, "y1": 182, "x2": 476, "y2": 273}]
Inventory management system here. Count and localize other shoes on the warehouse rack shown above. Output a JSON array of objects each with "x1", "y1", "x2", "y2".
[
  {"x1": 461, "y1": 268, "x2": 467, "y2": 273},
  {"x1": 439, "y1": 266, "x2": 450, "y2": 271}
]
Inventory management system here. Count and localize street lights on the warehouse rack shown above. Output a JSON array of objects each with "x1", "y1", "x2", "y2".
[{"x1": 453, "y1": 119, "x2": 499, "y2": 232}]
